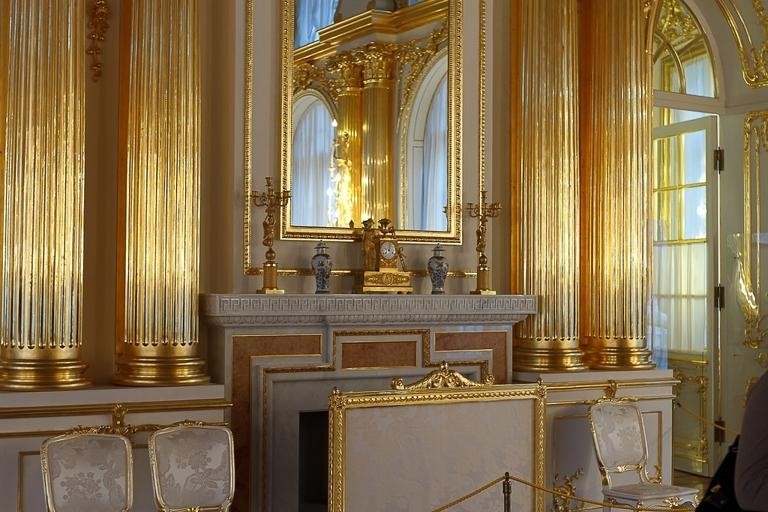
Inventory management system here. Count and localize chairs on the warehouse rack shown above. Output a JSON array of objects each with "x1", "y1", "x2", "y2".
[
  {"x1": 591, "y1": 398, "x2": 702, "y2": 511},
  {"x1": 39, "y1": 419, "x2": 235, "y2": 512}
]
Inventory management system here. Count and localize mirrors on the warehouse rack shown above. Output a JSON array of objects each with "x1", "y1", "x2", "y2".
[{"x1": 279, "y1": 0, "x2": 468, "y2": 247}]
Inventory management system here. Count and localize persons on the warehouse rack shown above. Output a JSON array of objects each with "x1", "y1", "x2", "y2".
[{"x1": 733, "y1": 367, "x2": 764, "y2": 512}]
